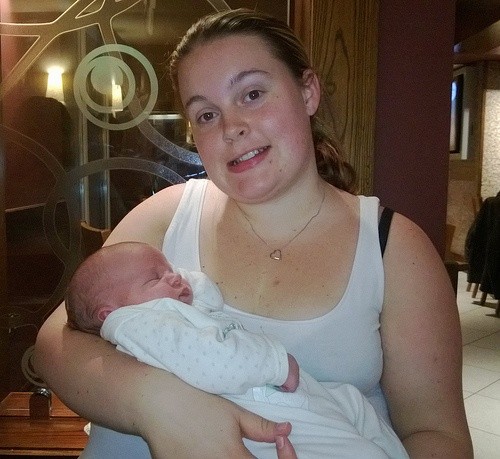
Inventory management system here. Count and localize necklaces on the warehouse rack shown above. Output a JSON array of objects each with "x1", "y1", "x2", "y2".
[{"x1": 231, "y1": 184, "x2": 329, "y2": 262}]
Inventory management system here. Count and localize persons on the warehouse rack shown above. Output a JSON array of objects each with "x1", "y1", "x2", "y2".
[
  {"x1": 64, "y1": 241, "x2": 409, "y2": 459},
  {"x1": 31, "y1": 9, "x2": 473, "y2": 459}
]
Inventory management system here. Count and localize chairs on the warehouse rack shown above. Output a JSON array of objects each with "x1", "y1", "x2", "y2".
[{"x1": 467, "y1": 196, "x2": 485, "y2": 297}]
